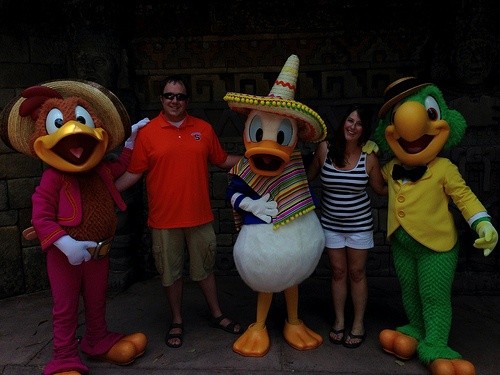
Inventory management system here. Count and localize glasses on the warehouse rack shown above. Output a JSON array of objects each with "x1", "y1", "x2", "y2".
[{"x1": 162, "y1": 92, "x2": 187, "y2": 100}]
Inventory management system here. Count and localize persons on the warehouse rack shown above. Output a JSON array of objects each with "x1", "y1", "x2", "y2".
[
  {"x1": 114, "y1": 73, "x2": 247, "y2": 349},
  {"x1": 306, "y1": 101, "x2": 389, "y2": 349}
]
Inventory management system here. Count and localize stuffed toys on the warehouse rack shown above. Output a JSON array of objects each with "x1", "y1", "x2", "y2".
[
  {"x1": 224, "y1": 54, "x2": 328, "y2": 359},
  {"x1": 0, "y1": 77, "x2": 149, "y2": 375},
  {"x1": 375, "y1": 74, "x2": 499, "y2": 375}
]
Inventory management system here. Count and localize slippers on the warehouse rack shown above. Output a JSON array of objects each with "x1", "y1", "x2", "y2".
[
  {"x1": 328, "y1": 325, "x2": 346, "y2": 344},
  {"x1": 343, "y1": 330, "x2": 366, "y2": 349}
]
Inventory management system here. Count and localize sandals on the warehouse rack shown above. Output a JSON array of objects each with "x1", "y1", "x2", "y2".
[
  {"x1": 208, "y1": 315, "x2": 243, "y2": 333},
  {"x1": 166, "y1": 323, "x2": 184, "y2": 348}
]
